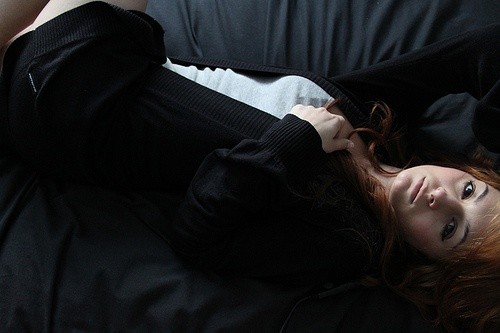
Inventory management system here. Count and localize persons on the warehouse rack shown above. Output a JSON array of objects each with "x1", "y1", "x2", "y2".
[{"x1": 1, "y1": 1, "x2": 500, "y2": 294}]
